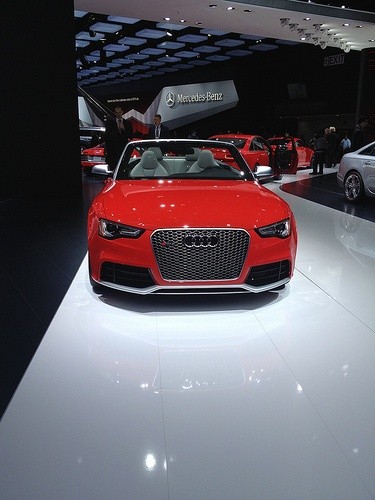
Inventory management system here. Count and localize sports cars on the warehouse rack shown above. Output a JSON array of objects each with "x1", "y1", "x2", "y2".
[{"x1": 87, "y1": 138, "x2": 297, "y2": 298}]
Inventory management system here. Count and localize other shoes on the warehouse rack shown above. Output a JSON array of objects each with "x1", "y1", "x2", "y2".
[
  {"x1": 324, "y1": 163, "x2": 337, "y2": 168},
  {"x1": 317, "y1": 172, "x2": 322, "y2": 174},
  {"x1": 309, "y1": 172, "x2": 314, "y2": 175}
]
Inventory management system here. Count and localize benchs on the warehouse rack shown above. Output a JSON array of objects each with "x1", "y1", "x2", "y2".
[{"x1": 148, "y1": 146, "x2": 201, "y2": 174}]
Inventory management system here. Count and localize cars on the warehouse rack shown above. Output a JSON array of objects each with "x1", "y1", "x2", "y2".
[
  {"x1": 267, "y1": 137, "x2": 315, "y2": 169},
  {"x1": 336, "y1": 141, "x2": 375, "y2": 204},
  {"x1": 80, "y1": 139, "x2": 149, "y2": 175},
  {"x1": 200, "y1": 134, "x2": 276, "y2": 175}
]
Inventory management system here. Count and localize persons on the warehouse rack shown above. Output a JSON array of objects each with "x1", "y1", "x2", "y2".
[
  {"x1": 148, "y1": 114, "x2": 170, "y2": 157},
  {"x1": 105, "y1": 107, "x2": 132, "y2": 168},
  {"x1": 272, "y1": 116, "x2": 368, "y2": 175}
]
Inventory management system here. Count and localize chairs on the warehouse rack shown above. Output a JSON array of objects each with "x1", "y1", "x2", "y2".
[
  {"x1": 130, "y1": 151, "x2": 168, "y2": 176},
  {"x1": 187, "y1": 149, "x2": 220, "y2": 173}
]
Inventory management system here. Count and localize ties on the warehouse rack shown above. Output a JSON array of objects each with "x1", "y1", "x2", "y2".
[
  {"x1": 118, "y1": 119, "x2": 122, "y2": 129},
  {"x1": 156, "y1": 126, "x2": 159, "y2": 137}
]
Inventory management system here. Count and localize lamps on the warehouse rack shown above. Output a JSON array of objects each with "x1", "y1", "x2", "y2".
[{"x1": 282, "y1": 19, "x2": 351, "y2": 53}]
